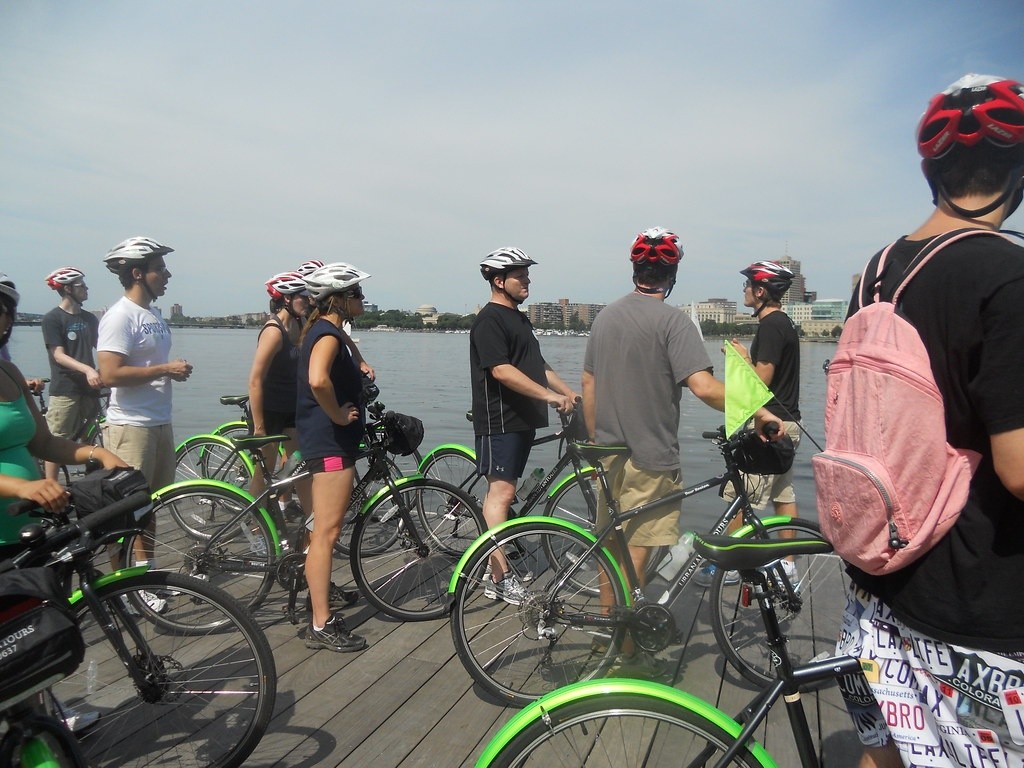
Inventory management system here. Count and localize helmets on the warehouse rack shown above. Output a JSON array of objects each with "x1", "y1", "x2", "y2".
[
  {"x1": 103, "y1": 237, "x2": 175, "y2": 274},
  {"x1": 739, "y1": 260, "x2": 795, "y2": 299},
  {"x1": 629, "y1": 226, "x2": 685, "y2": 265},
  {"x1": 44, "y1": 267, "x2": 86, "y2": 290},
  {"x1": 0, "y1": 273, "x2": 20, "y2": 313},
  {"x1": 304, "y1": 263, "x2": 372, "y2": 303},
  {"x1": 479, "y1": 247, "x2": 537, "y2": 281},
  {"x1": 913, "y1": 72, "x2": 1024, "y2": 160},
  {"x1": 265, "y1": 272, "x2": 311, "y2": 300},
  {"x1": 298, "y1": 260, "x2": 322, "y2": 278}
]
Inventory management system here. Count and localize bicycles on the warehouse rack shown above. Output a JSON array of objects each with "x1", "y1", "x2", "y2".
[
  {"x1": 0, "y1": 491, "x2": 278, "y2": 768},
  {"x1": 440, "y1": 422, "x2": 835, "y2": 708},
  {"x1": 30, "y1": 375, "x2": 672, "y2": 597},
  {"x1": 120, "y1": 400, "x2": 489, "y2": 637},
  {"x1": 474, "y1": 533, "x2": 864, "y2": 768}
]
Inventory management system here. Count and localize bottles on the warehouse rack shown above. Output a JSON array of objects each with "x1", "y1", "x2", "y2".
[
  {"x1": 277, "y1": 450, "x2": 302, "y2": 480},
  {"x1": 300, "y1": 510, "x2": 314, "y2": 555},
  {"x1": 280, "y1": 533, "x2": 290, "y2": 550},
  {"x1": 655, "y1": 530, "x2": 699, "y2": 580},
  {"x1": 515, "y1": 468, "x2": 546, "y2": 501}
]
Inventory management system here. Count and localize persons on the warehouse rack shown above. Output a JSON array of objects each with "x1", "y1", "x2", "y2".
[
  {"x1": 296, "y1": 263, "x2": 372, "y2": 652},
  {"x1": 835, "y1": 73, "x2": 1024, "y2": 767},
  {"x1": 469, "y1": 247, "x2": 582, "y2": 605},
  {"x1": 248, "y1": 258, "x2": 325, "y2": 557},
  {"x1": 43, "y1": 266, "x2": 99, "y2": 482},
  {"x1": 694, "y1": 261, "x2": 803, "y2": 588},
  {"x1": 95, "y1": 236, "x2": 186, "y2": 613},
  {"x1": 0, "y1": 275, "x2": 129, "y2": 732},
  {"x1": 581, "y1": 227, "x2": 784, "y2": 678}
]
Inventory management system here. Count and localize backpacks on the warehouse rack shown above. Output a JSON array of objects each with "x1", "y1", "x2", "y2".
[{"x1": 812, "y1": 228, "x2": 1016, "y2": 577}]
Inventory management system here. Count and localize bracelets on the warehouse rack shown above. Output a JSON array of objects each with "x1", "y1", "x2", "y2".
[
  {"x1": 256, "y1": 427, "x2": 265, "y2": 430},
  {"x1": 89, "y1": 443, "x2": 98, "y2": 463}
]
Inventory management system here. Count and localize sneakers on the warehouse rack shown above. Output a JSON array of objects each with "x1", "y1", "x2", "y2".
[
  {"x1": 692, "y1": 563, "x2": 742, "y2": 587},
  {"x1": 608, "y1": 648, "x2": 665, "y2": 679},
  {"x1": 153, "y1": 588, "x2": 181, "y2": 594},
  {"x1": 753, "y1": 567, "x2": 797, "y2": 591},
  {"x1": 52, "y1": 700, "x2": 100, "y2": 733},
  {"x1": 107, "y1": 588, "x2": 168, "y2": 616},
  {"x1": 305, "y1": 582, "x2": 359, "y2": 611},
  {"x1": 478, "y1": 568, "x2": 534, "y2": 582},
  {"x1": 305, "y1": 612, "x2": 366, "y2": 652},
  {"x1": 590, "y1": 630, "x2": 617, "y2": 656},
  {"x1": 484, "y1": 573, "x2": 529, "y2": 606}
]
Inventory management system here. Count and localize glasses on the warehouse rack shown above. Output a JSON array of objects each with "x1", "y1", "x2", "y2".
[
  {"x1": 70, "y1": 282, "x2": 87, "y2": 289},
  {"x1": 743, "y1": 282, "x2": 755, "y2": 289},
  {"x1": 335, "y1": 287, "x2": 363, "y2": 298}
]
